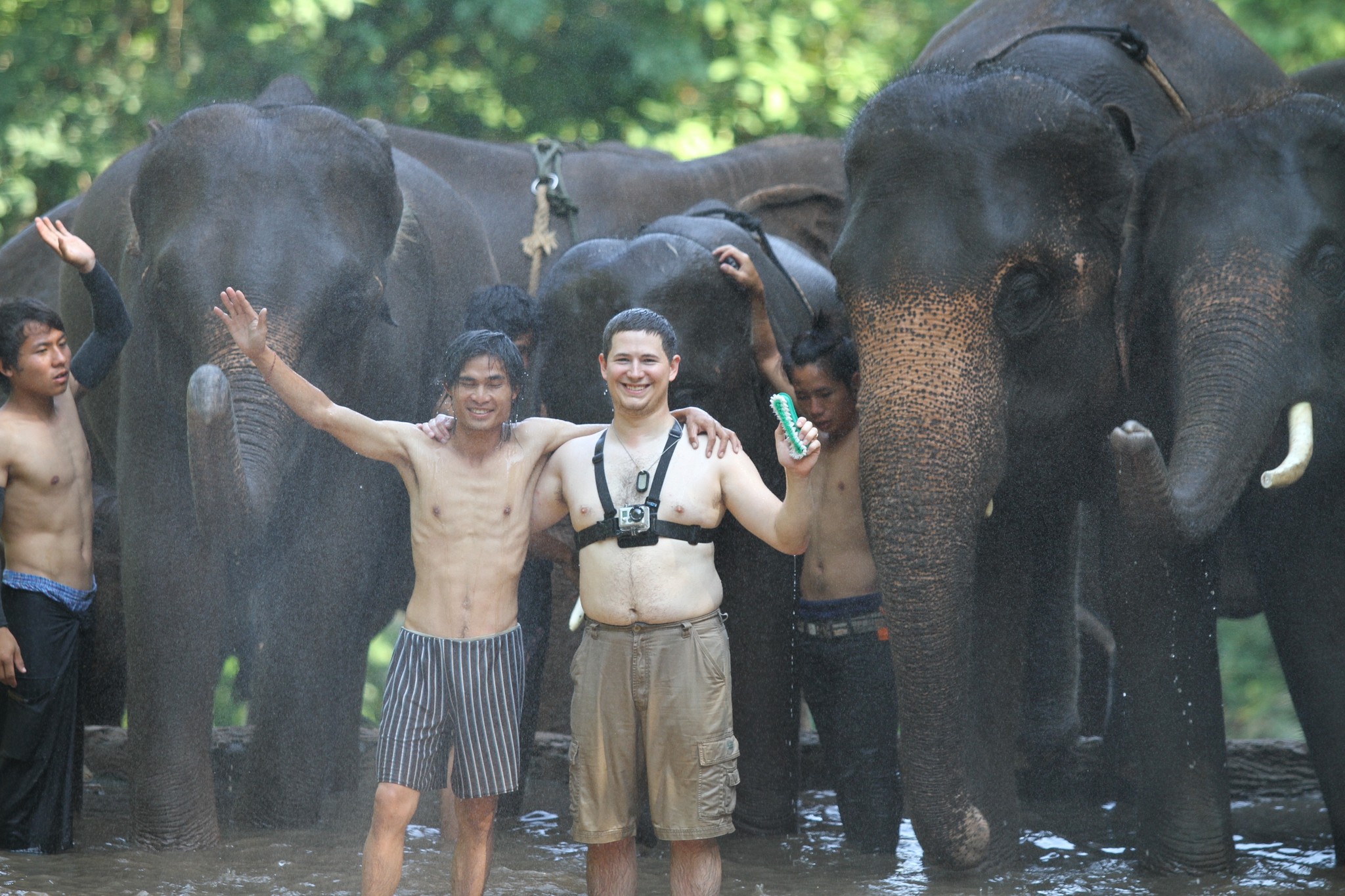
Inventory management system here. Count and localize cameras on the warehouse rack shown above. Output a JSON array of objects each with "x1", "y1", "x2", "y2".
[{"x1": 619, "y1": 505, "x2": 650, "y2": 533}]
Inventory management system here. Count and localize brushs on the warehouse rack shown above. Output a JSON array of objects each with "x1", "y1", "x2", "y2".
[{"x1": 770, "y1": 392, "x2": 807, "y2": 461}]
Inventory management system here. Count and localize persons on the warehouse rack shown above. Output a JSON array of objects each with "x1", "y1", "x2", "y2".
[
  {"x1": 431, "y1": 283, "x2": 581, "y2": 854},
  {"x1": 214, "y1": 287, "x2": 740, "y2": 896},
  {"x1": 712, "y1": 244, "x2": 994, "y2": 855},
  {"x1": 0, "y1": 216, "x2": 132, "y2": 854},
  {"x1": 416, "y1": 307, "x2": 821, "y2": 896}
]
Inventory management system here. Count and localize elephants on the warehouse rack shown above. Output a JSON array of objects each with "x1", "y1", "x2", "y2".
[{"x1": 0, "y1": 0, "x2": 1344, "y2": 887}]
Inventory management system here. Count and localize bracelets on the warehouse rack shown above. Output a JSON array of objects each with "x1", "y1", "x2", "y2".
[{"x1": 264, "y1": 347, "x2": 277, "y2": 383}]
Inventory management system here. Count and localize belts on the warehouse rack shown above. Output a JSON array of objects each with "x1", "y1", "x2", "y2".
[{"x1": 795, "y1": 612, "x2": 889, "y2": 639}]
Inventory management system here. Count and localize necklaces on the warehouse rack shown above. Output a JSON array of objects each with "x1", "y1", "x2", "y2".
[{"x1": 611, "y1": 416, "x2": 682, "y2": 492}]
